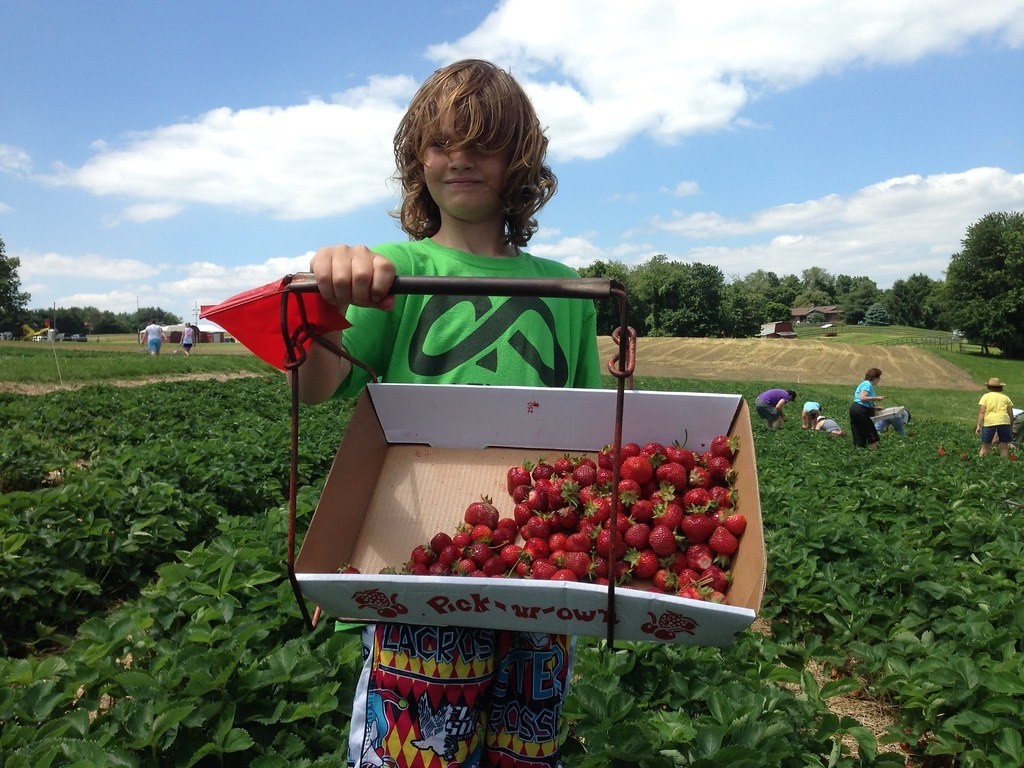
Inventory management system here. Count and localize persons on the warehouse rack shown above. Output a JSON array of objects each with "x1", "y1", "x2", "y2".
[
  {"x1": 802, "y1": 401, "x2": 845, "y2": 437},
  {"x1": 179, "y1": 323, "x2": 195, "y2": 357},
  {"x1": 755, "y1": 389, "x2": 797, "y2": 429},
  {"x1": 284, "y1": 59, "x2": 605, "y2": 768},
  {"x1": 875, "y1": 409, "x2": 910, "y2": 437},
  {"x1": 976, "y1": 378, "x2": 1024, "y2": 457},
  {"x1": 849, "y1": 368, "x2": 885, "y2": 451},
  {"x1": 141, "y1": 319, "x2": 168, "y2": 356}
]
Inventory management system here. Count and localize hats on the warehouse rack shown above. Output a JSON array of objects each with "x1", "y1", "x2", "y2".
[
  {"x1": 816, "y1": 416, "x2": 826, "y2": 422},
  {"x1": 984, "y1": 377, "x2": 1007, "y2": 387}
]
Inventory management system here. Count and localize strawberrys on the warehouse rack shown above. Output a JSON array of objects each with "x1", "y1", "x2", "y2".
[
  {"x1": 378, "y1": 437, "x2": 745, "y2": 604},
  {"x1": 337, "y1": 564, "x2": 360, "y2": 574}
]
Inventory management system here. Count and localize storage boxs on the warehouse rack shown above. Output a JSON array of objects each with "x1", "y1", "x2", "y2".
[
  {"x1": 870, "y1": 405, "x2": 904, "y2": 421},
  {"x1": 288, "y1": 382, "x2": 769, "y2": 644}
]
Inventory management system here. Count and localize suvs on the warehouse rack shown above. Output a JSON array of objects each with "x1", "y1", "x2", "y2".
[
  {"x1": 70, "y1": 333, "x2": 89, "y2": 343},
  {"x1": 54, "y1": 332, "x2": 71, "y2": 342}
]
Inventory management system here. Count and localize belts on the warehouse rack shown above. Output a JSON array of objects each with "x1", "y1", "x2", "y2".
[{"x1": 1013, "y1": 412, "x2": 1024, "y2": 421}]
